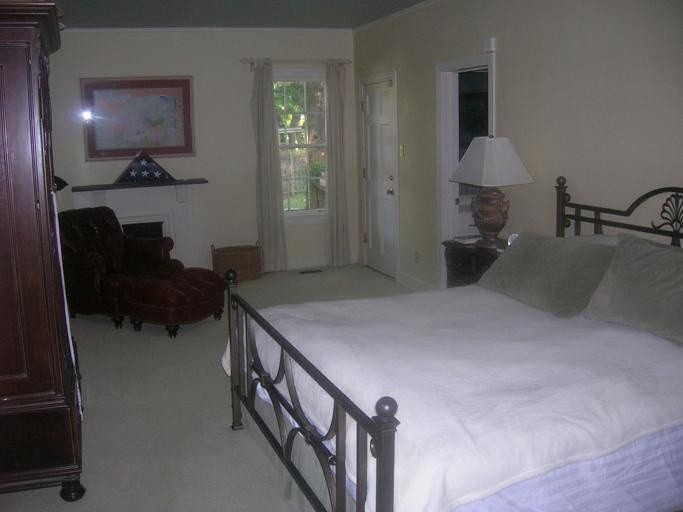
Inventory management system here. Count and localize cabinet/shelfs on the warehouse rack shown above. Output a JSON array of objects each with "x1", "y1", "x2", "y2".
[{"x1": 0, "y1": 1, "x2": 85, "y2": 502}]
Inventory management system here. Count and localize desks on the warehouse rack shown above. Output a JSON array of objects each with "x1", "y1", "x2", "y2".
[{"x1": 440, "y1": 235, "x2": 507, "y2": 288}]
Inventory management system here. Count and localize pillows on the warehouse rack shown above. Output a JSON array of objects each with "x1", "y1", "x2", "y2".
[{"x1": 475, "y1": 231, "x2": 682, "y2": 347}]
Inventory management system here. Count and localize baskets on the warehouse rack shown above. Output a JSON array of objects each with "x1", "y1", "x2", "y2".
[{"x1": 211, "y1": 240, "x2": 262, "y2": 282}]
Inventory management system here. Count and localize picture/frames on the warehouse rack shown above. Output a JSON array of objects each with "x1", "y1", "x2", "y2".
[{"x1": 79, "y1": 76, "x2": 196, "y2": 162}]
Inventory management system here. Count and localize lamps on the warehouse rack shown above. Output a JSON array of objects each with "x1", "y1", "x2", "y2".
[{"x1": 447, "y1": 136, "x2": 534, "y2": 249}]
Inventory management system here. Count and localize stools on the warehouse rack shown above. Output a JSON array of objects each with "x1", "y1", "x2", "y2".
[{"x1": 127, "y1": 267, "x2": 227, "y2": 338}]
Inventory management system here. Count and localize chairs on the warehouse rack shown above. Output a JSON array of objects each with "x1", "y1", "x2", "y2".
[{"x1": 57, "y1": 206, "x2": 184, "y2": 330}]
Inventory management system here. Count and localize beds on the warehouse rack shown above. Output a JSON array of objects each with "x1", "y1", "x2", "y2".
[{"x1": 223, "y1": 176, "x2": 682, "y2": 511}]
